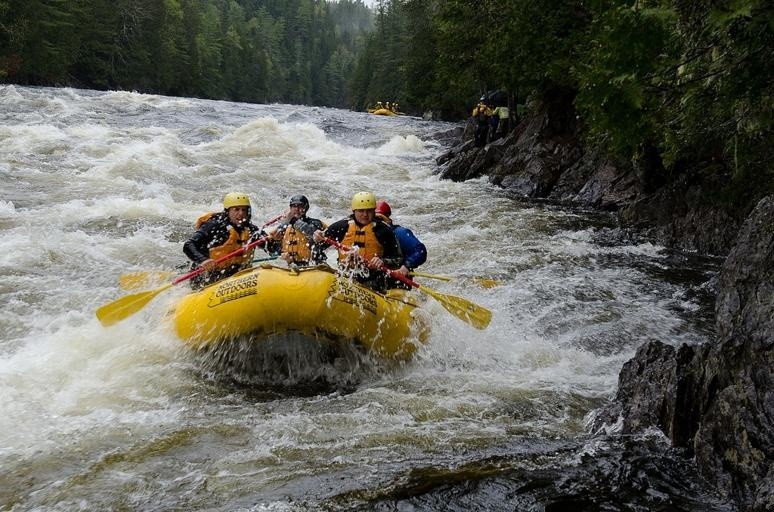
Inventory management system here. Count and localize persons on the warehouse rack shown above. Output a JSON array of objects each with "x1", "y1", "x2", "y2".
[
  {"x1": 375, "y1": 201, "x2": 427, "y2": 295},
  {"x1": 183, "y1": 191, "x2": 284, "y2": 289},
  {"x1": 313, "y1": 192, "x2": 404, "y2": 295},
  {"x1": 374, "y1": 101, "x2": 401, "y2": 114},
  {"x1": 471, "y1": 97, "x2": 518, "y2": 147},
  {"x1": 255, "y1": 194, "x2": 335, "y2": 267}
]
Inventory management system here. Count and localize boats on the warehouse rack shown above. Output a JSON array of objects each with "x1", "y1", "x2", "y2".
[
  {"x1": 173, "y1": 262, "x2": 432, "y2": 363},
  {"x1": 374, "y1": 109, "x2": 396, "y2": 116}
]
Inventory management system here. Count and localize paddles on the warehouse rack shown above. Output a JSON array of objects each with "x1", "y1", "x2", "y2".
[
  {"x1": 118, "y1": 271, "x2": 192, "y2": 293},
  {"x1": 97, "y1": 236, "x2": 271, "y2": 327},
  {"x1": 408, "y1": 271, "x2": 502, "y2": 290},
  {"x1": 322, "y1": 234, "x2": 492, "y2": 331}
]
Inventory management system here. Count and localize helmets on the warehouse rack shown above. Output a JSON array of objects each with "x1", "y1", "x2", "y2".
[
  {"x1": 352, "y1": 192, "x2": 392, "y2": 213},
  {"x1": 224, "y1": 192, "x2": 252, "y2": 208},
  {"x1": 290, "y1": 195, "x2": 309, "y2": 205}
]
